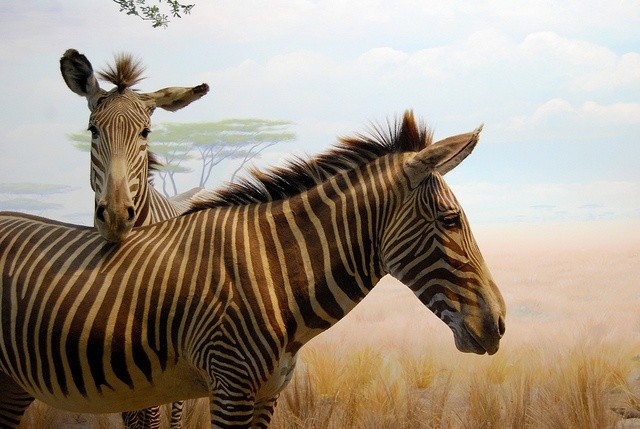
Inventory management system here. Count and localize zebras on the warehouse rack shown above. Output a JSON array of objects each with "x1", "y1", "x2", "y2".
[
  {"x1": 59, "y1": 49, "x2": 225, "y2": 428},
  {"x1": 0, "y1": 111, "x2": 506, "y2": 429}
]
